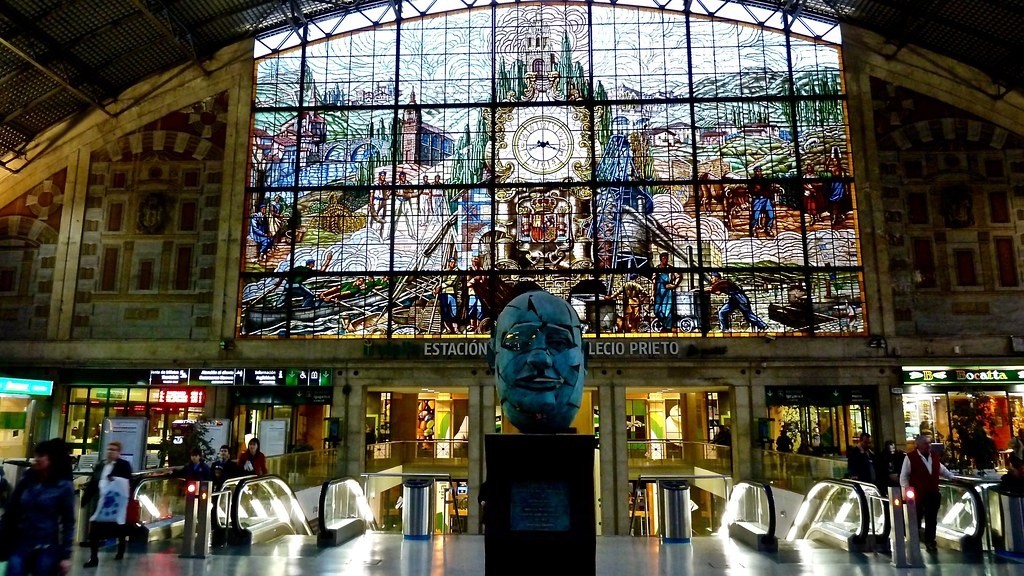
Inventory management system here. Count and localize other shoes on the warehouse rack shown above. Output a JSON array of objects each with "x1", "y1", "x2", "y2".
[
  {"x1": 83, "y1": 555, "x2": 98, "y2": 568},
  {"x1": 925, "y1": 541, "x2": 937, "y2": 551},
  {"x1": 116, "y1": 552, "x2": 123, "y2": 560}
]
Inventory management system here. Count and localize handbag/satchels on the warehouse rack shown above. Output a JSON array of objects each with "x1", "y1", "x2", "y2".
[
  {"x1": 126, "y1": 488, "x2": 140, "y2": 525},
  {"x1": 89, "y1": 476, "x2": 129, "y2": 525},
  {"x1": 0, "y1": 480, "x2": 27, "y2": 562}
]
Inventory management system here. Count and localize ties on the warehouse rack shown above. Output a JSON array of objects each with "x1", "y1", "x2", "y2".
[
  {"x1": 922, "y1": 451, "x2": 928, "y2": 462},
  {"x1": 863, "y1": 450, "x2": 869, "y2": 464}
]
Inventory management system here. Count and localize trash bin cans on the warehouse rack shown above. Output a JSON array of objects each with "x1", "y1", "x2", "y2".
[
  {"x1": 659, "y1": 480, "x2": 692, "y2": 544},
  {"x1": 402, "y1": 478, "x2": 431, "y2": 540},
  {"x1": 987, "y1": 486, "x2": 1024, "y2": 553}
]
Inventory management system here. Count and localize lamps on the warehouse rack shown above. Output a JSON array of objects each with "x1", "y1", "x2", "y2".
[
  {"x1": 867, "y1": 334, "x2": 886, "y2": 348},
  {"x1": 220, "y1": 338, "x2": 237, "y2": 351}
]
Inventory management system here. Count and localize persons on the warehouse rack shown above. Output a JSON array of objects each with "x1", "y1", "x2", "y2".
[
  {"x1": 0, "y1": 438, "x2": 268, "y2": 576},
  {"x1": 712, "y1": 425, "x2": 1024, "y2": 564}
]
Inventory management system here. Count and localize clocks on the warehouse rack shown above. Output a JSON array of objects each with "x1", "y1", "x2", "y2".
[{"x1": 514, "y1": 116, "x2": 574, "y2": 172}]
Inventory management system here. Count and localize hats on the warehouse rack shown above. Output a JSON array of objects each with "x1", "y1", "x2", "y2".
[{"x1": 215, "y1": 462, "x2": 224, "y2": 471}]
